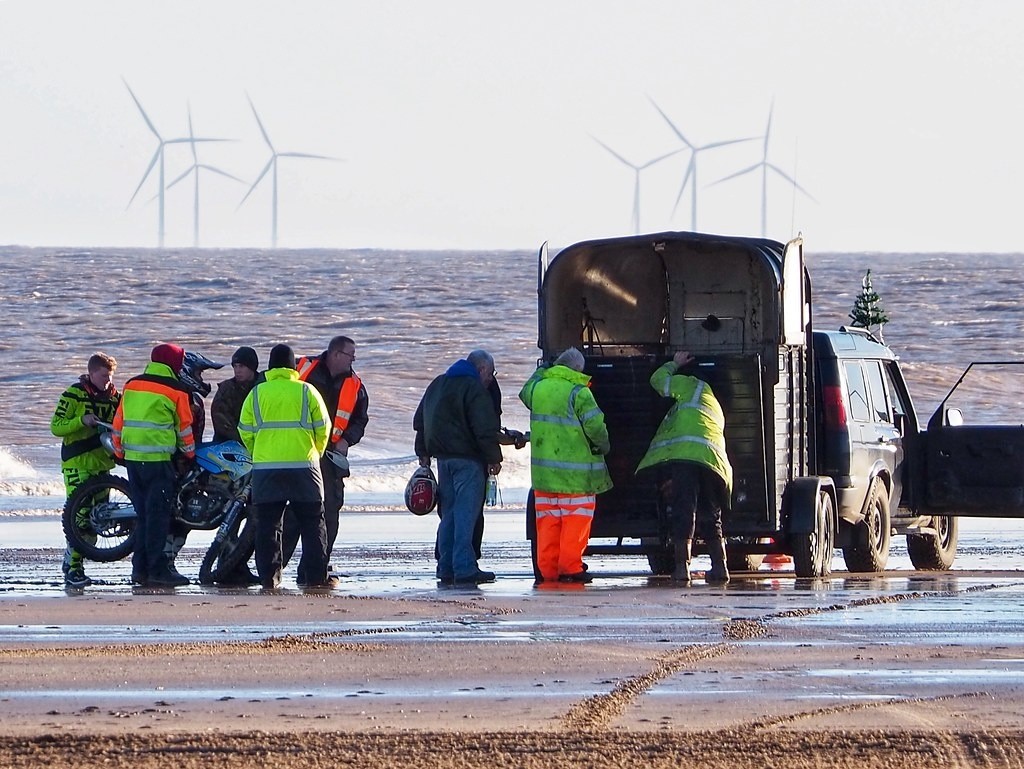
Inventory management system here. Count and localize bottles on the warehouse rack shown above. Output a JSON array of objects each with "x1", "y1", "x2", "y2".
[{"x1": 486, "y1": 469, "x2": 497, "y2": 506}]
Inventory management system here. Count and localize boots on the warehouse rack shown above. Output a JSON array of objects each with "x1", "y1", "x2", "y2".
[
  {"x1": 704, "y1": 538, "x2": 732, "y2": 583},
  {"x1": 670, "y1": 539, "x2": 693, "y2": 581}
]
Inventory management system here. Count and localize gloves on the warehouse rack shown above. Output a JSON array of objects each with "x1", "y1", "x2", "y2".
[
  {"x1": 109, "y1": 453, "x2": 126, "y2": 466},
  {"x1": 180, "y1": 454, "x2": 198, "y2": 469}
]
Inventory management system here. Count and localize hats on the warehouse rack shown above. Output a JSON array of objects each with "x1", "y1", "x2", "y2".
[
  {"x1": 553, "y1": 348, "x2": 585, "y2": 372},
  {"x1": 266, "y1": 342, "x2": 297, "y2": 368},
  {"x1": 231, "y1": 346, "x2": 262, "y2": 371}
]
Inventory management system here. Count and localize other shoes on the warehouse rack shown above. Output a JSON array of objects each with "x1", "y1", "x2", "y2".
[
  {"x1": 64, "y1": 569, "x2": 94, "y2": 586},
  {"x1": 299, "y1": 574, "x2": 341, "y2": 597},
  {"x1": 244, "y1": 573, "x2": 262, "y2": 586},
  {"x1": 581, "y1": 563, "x2": 590, "y2": 571},
  {"x1": 558, "y1": 571, "x2": 594, "y2": 583},
  {"x1": 129, "y1": 571, "x2": 149, "y2": 586},
  {"x1": 532, "y1": 580, "x2": 545, "y2": 588},
  {"x1": 61, "y1": 563, "x2": 86, "y2": 574},
  {"x1": 145, "y1": 572, "x2": 191, "y2": 588},
  {"x1": 454, "y1": 570, "x2": 496, "y2": 584},
  {"x1": 438, "y1": 575, "x2": 455, "y2": 583}
]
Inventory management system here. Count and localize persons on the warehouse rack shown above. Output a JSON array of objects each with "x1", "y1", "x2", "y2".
[
  {"x1": 518, "y1": 346, "x2": 613, "y2": 582},
  {"x1": 413, "y1": 350, "x2": 527, "y2": 590},
  {"x1": 634, "y1": 350, "x2": 734, "y2": 583},
  {"x1": 50, "y1": 351, "x2": 122, "y2": 593},
  {"x1": 111, "y1": 343, "x2": 226, "y2": 587},
  {"x1": 282, "y1": 336, "x2": 369, "y2": 580},
  {"x1": 211, "y1": 344, "x2": 339, "y2": 591}
]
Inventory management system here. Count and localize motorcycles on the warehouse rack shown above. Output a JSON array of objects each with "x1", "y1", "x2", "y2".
[{"x1": 60, "y1": 419, "x2": 263, "y2": 585}]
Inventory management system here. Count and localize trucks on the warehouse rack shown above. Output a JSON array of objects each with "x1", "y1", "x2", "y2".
[{"x1": 529, "y1": 226, "x2": 1024, "y2": 584}]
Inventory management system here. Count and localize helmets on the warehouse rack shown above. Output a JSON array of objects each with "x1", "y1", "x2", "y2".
[
  {"x1": 403, "y1": 464, "x2": 438, "y2": 517},
  {"x1": 178, "y1": 351, "x2": 224, "y2": 397}
]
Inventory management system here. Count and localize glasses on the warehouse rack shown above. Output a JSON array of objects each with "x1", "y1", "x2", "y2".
[
  {"x1": 338, "y1": 350, "x2": 357, "y2": 360},
  {"x1": 492, "y1": 370, "x2": 499, "y2": 377}
]
